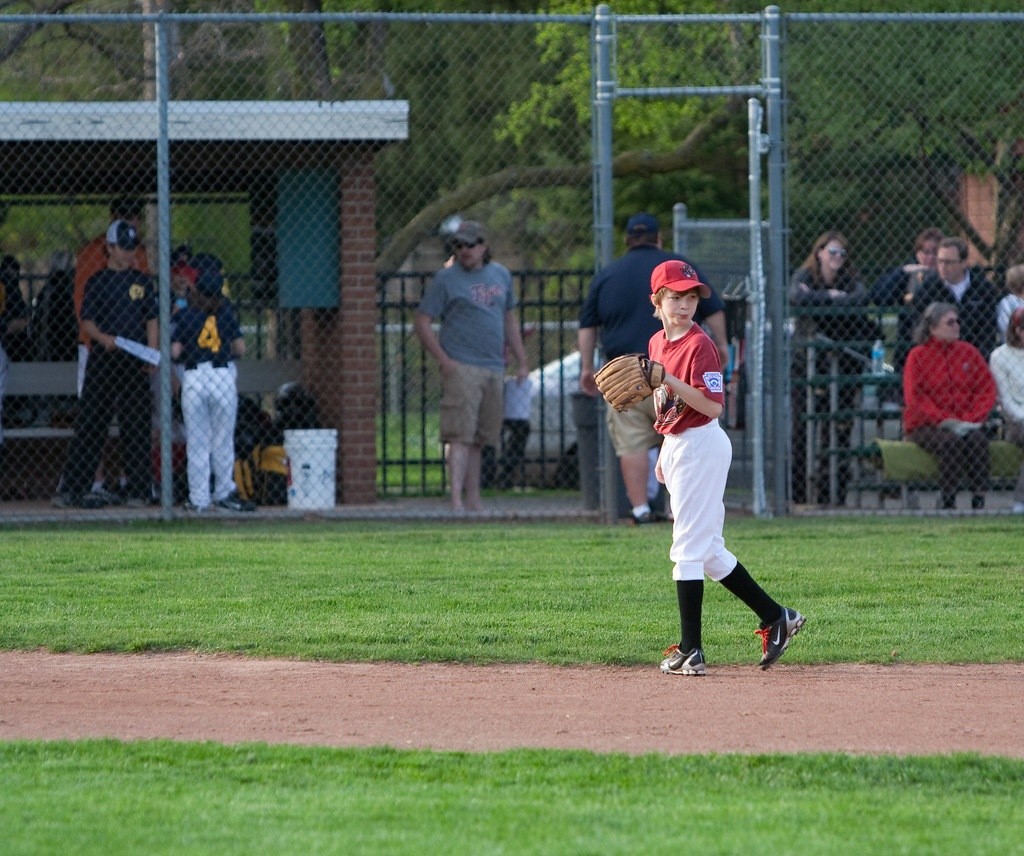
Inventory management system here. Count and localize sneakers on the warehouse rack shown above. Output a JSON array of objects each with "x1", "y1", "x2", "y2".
[
  {"x1": 754, "y1": 606, "x2": 806, "y2": 671},
  {"x1": 660, "y1": 644, "x2": 706, "y2": 676}
]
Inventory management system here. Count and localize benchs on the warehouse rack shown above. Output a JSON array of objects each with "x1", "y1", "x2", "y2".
[
  {"x1": 869, "y1": 437, "x2": 1023, "y2": 510},
  {"x1": 0, "y1": 358, "x2": 162, "y2": 489}
]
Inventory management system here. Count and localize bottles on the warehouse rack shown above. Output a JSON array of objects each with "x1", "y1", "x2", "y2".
[
  {"x1": 175, "y1": 291, "x2": 188, "y2": 307},
  {"x1": 871, "y1": 339, "x2": 885, "y2": 375}
]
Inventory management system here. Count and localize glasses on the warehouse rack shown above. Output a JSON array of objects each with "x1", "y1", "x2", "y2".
[
  {"x1": 934, "y1": 317, "x2": 960, "y2": 326},
  {"x1": 456, "y1": 241, "x2": 482, "y2": 249},
  {"x1": 822, "y1": 247, "x2": 847, "y2": 258}
]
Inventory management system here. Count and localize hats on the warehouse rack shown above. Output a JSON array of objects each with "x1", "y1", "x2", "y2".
[
  {"x1": 454, "y1": 220, "x2": 487, "y2": 245},
  {"x1": 105, "y1": 219, "x2": 139, "y2": 250},
  {"x1": 650, "y1": 259, "x2": 711, "y2": 299},
  {"x1": 627, "y1": 214, "x2": 659, "y2": 233}
]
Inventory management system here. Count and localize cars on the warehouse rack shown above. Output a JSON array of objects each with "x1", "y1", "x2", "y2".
[{"x1": 443, "y1": 348, "x2": 778, "y2": 493}]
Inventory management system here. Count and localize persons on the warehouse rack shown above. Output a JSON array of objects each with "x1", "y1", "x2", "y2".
[
  {"x1": 869, "y1": 227, "x2": 1024, "y2": 441},
  {"x1": 989, "y1": 307, "x2": 1024, "y2": 512},
  {"x1": 902, "y1": 302, "x2": 997, "y2": 510},
  {"x1": 789, "y1": 230, "x2": 869, "y2": 509},
  {"x1": 576, "y1": 210, "x2": 729, "y2": 524},
  {"x1": 0, "y1": 197, "x2": 256, "y2": 513},
  {"x1": 415, "y1": 220, "x2": 526, "y2": 512},
  {"x1": 594, "y1": 259, "x2": 806, "y2": 676}
]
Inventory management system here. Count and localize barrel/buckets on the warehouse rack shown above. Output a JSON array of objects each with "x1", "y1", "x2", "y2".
[{"x1": 283, "y1": 428, "x2": 339, "y2": 510}]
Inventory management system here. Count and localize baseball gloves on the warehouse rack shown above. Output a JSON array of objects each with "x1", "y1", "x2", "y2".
[{"x1": 592, "y1": 352, "x2": 666, "y2": 412}]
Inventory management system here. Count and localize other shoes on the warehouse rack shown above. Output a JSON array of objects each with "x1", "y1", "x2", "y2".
[
  {"x1": 50, "y1": 491, "x2": 85, "y2": 507},
  {"x1": 942, "y1": 494, "x2": 956, "y2": 510},
  {"x1": 126, "y1": 489, "x2": 152, "y2": 507},
  {"x1": 1011, "y1": 498, "x2": 1024, "y2": 517},
  {"x1": 971, "y1": 494, "x2": 985, "y2": 508},
  {"x1": 184, "y1": 502, "x2": 217, "y2": 513},
  {"x1": 631, "y1": 506, "x2": 653, "y2": 523},
  {"x1": 656, "y1": 509, "x2": 675, "y2": 523},
  {"x1": 215, "y1": 495, "x2": 257, "y2": 511}
]
